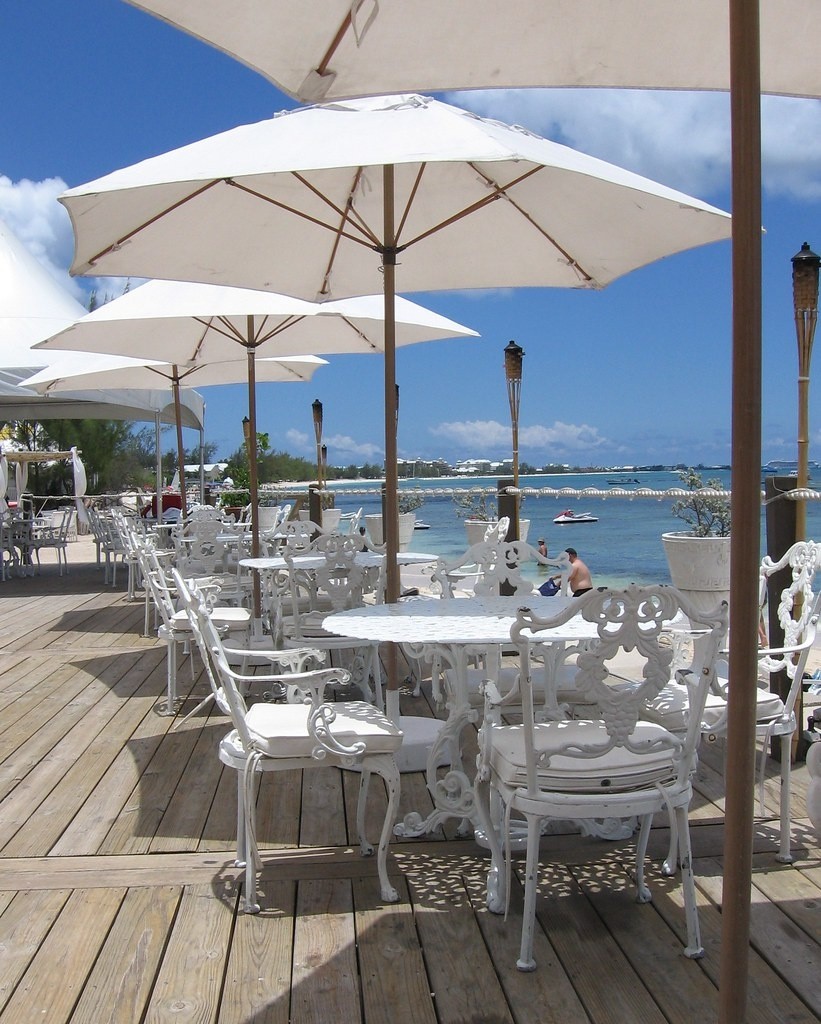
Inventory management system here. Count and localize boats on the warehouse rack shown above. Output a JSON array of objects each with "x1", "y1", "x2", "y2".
[
  {"x1": 787, "y1": 469, "x2": 800, "y2": 479},
  {"x1": 413, "y1": 519, "x2": 431, "y2": 529},
  {"x1": 552, "y1": 509, "x2": 600, "y2": 523},
  {"x1": 605, "y1": 477, "x2": 640, "y2": 486}
]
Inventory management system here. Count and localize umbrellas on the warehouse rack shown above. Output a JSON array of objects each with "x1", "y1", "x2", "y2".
[
  {"x1": 59, "y1": 95, "x2": 763, "y2": 725},
  {"x1": 30, "y1": 278, "x2": 480, "y2": 650},
  {"x1": 18, "y1": 352, "x2": 328, "y2": 557},
  {"x1": 127, "y1": 0, "x2": 818, "y2": 751}
]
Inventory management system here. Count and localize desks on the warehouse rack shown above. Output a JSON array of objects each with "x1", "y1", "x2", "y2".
[
  {"x1": 179, "y1": 531, "x2": 313, "y2": 573},
  {"x1": 0, "y1": 518, "x2": 44, "y2": 523},
  {"x1": 150, "y1": 522, "x2": 252, "y2": 536},
  {"x1": 238, "y1": 550, "x2": 439, "y2": 701},
  {"x1": 34, "y1": 492, "x2": 182, "y2": 517},
  {"x1": 321, "y1": 596, "x2": 683, "y2": 914}
]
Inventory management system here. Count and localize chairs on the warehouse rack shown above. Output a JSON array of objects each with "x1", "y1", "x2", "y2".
[{"x1": 0, "y1": 505, "x2": 821, "y2": 974}]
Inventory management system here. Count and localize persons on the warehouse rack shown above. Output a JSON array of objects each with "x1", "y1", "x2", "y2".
[
  {"x1": 536, "y1": 538, "x2": 547, "y2": 566},
  {"x1": 551, "y1": 548, "x2": 593, "y2": 597}
]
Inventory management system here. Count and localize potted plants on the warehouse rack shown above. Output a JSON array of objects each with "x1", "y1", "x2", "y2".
[
  {"x1": 298, "y1": 492, "x2": 341, "y2": 531},
  {"x1": 257, "y1": 485, "x2": 287, "y2": 530},
  {"x1": 450, "y1": 487, "x2": 531, "y2": 546},
  {"x1": 660, "y1": 467, "x2": 731, "y2": 592},
  {"x1": 364, "y1": 489, "x2": 426, "y2": 551},
  {"x1": 219, "y1": 492, "x2": 250, "y2": 523}
]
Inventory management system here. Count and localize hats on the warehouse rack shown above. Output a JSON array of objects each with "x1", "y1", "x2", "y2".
[{"x1": 537, "y1": 537, "x2": 545, "y2": 543}]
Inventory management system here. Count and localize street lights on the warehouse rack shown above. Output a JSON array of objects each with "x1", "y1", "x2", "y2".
[
  {"x1": 310, "y1": 397, "x2": 326, "y2": 518},
  {"x1": 779, "y1": 241, "x2": 821, "y2": 765},
  {"x1": 241, "y1": 415, "x2": 252, "y2": 494},
  {"x1": 500, "y1": 339, "x2": 525, "y2": 545}
]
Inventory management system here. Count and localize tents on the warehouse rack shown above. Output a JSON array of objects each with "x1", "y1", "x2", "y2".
[{"x1": 0, "y1": 219, "x2": 207, "y2": 535}]
